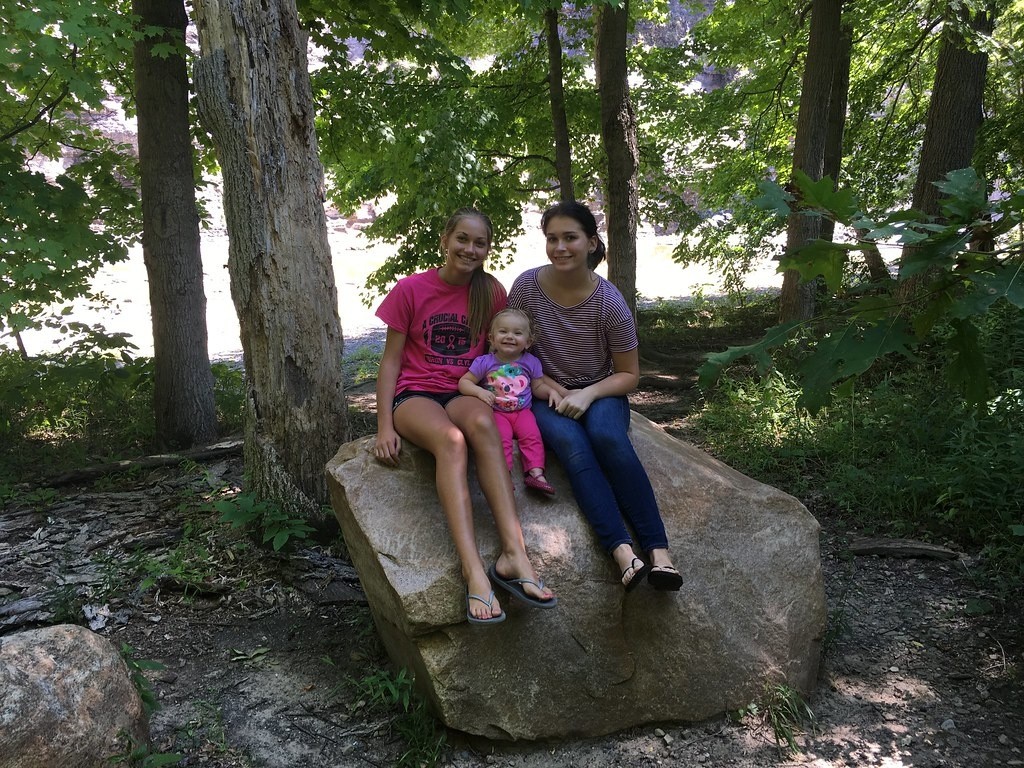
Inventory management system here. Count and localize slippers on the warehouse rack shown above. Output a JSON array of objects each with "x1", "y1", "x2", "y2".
[
  {"x1": 648, "y1": 565, "x2": 683, "y2": 591},
  {"x1": 621, "y1": 558, "x2": 648, "y2": 593},
  {"x1": 465, "y1": 583, "x2": 506, "y2": 625},
  {"x1": 489, "y1": 564, "x2": 558, "y2": 609}
]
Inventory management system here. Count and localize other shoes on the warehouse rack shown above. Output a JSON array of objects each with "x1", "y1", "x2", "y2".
[{"x1": 524, "y1": 474, "x2": 555, "y2": 494}]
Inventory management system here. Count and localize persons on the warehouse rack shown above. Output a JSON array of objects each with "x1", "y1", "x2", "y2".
[
  {"x1": 504, "y1": 202, "x2": 684, "y2": 591},
  {"x1": 374, "y1": 207, "x2": 557, "y2": 624},
  {"x1": 458, "y1": 307, "x2": 563, "y2": 495}
]
